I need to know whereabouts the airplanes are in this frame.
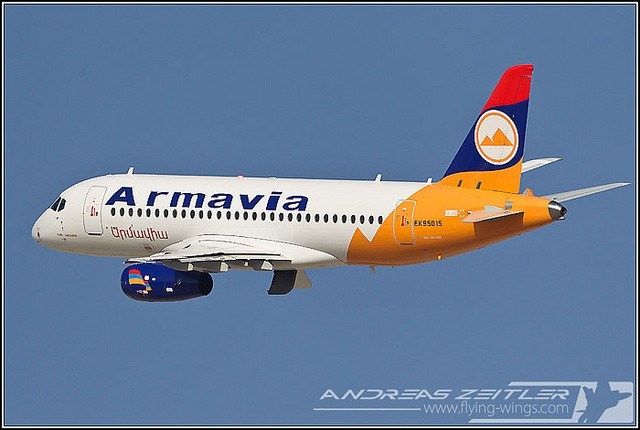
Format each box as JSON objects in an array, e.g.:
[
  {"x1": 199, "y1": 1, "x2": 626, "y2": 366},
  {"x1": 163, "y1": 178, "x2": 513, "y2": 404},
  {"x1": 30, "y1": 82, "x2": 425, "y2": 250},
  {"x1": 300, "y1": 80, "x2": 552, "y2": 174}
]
[{"x1": 31, "y1": 63, "x2": 631, "y2": 302}]
